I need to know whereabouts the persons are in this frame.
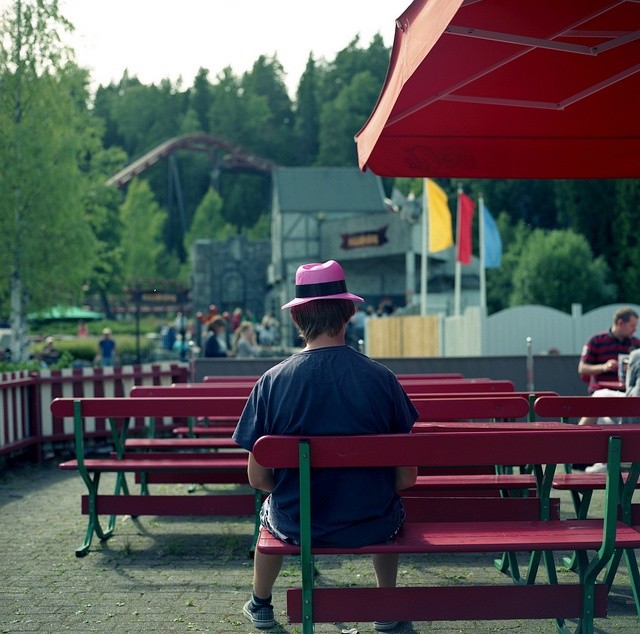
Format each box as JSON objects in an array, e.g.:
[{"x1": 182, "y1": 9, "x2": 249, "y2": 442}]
[
  {"x1": 579, "y1": 349, "x2": 640, "y2": 424},
  {"x1": 233, "y1": 322, "x2": 260, "y2": 358},
  {"x1": 352, "y1": 300, "x2": 406, "y2": 331},
  {"x1": 40, "y1": 336, "x2": 62, "y2": 364},
  {"x1": 579, "y1": 308, "x2": 640, "y2": 426},
  {"x1": 98, "y1": 328, "x2": 118, "y2": 366},
  {"x1": 232, "y1": 260, "x2": 421, "y2": 631},
  {"x1": 619, "y1": 349, "x2": 640, "y2": 425},
  {"x1": 206, "y1": 316, "x2": 235, "y2": 357}
]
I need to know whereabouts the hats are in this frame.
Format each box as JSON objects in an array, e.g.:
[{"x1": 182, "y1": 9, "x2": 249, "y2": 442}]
[{"x1": 281, "y1": 259, "x2": 365, "y2": 310}]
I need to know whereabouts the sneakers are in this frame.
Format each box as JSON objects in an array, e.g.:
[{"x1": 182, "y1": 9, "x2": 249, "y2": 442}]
[
  {"x1": 374, "y1": 620, "x2": 401, "y2": 630},
  {"x1": 242, "y1": 600, "x2": 276, "y2": 628},
  {"x1": 585, "y1": 463, "x2": 606, "y2": 473}
]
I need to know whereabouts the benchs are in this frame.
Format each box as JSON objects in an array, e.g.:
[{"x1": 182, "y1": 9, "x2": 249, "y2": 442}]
[
  {"x1": 203, "y1": 373, "x2": 465, "y2": 455},
  {"x1": 253, "y1": 427, "x2": 640, "y2": 633},
  {"x1": 168, "y1": 380, "x2": 519, "y2": 494},
  {"x1": 49, "y1": 395, "x2": 533, "y2": 586},
  {"x1": 129, "y1": 386, "x2": 560, "y2": 521},
  {"x1": 534, "y1": 395, "x2": 640, "y2": 573}
]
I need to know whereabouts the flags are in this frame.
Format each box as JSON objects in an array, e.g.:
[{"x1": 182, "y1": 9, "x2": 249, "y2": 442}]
[
  {"x1": 457, "y1": 194, "x2": 476, "y2": 265},
  {"x1": 483, "y1": 205, "x2": 503, "y2": 269},
  {"x1": 425, "y1": 177, "x2": 454, "y2": 254}
]
